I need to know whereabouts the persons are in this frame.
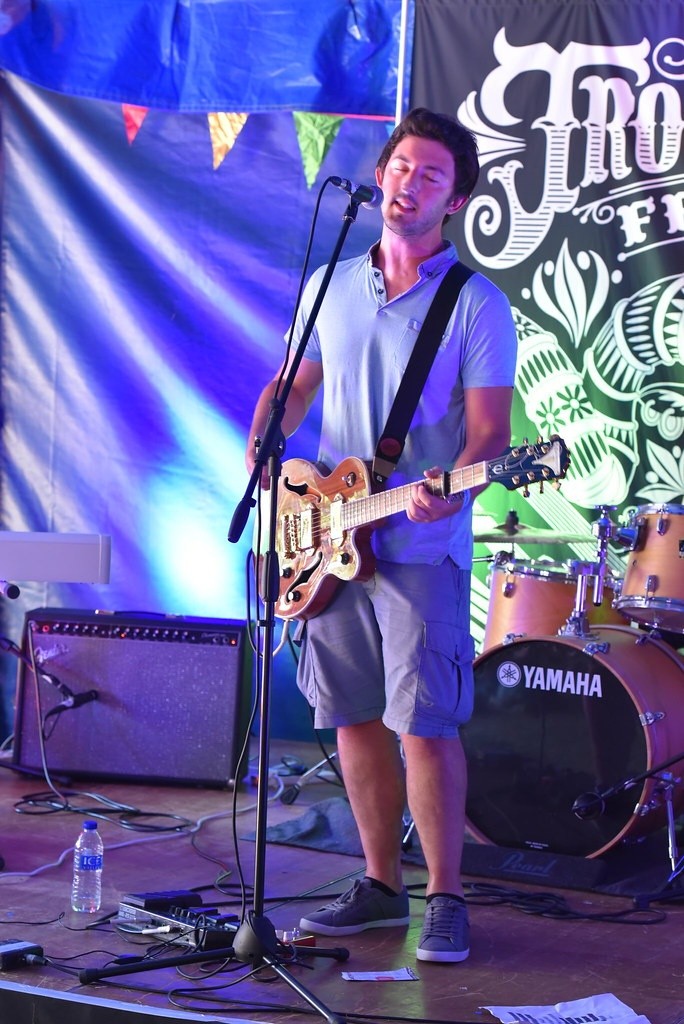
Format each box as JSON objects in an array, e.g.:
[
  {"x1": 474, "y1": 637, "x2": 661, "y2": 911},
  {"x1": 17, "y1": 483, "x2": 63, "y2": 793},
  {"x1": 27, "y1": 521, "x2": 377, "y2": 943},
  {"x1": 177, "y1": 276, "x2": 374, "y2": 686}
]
[{"x1": 247, "y1": 106, "x2": 518, "y2": 960}]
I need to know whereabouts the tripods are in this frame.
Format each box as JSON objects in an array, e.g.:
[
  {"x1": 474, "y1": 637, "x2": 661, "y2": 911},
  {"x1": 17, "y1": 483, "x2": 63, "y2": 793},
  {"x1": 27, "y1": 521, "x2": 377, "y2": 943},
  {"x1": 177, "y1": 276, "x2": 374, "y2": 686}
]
[{"x1": 77, "y1": 202, "x2": 350, "y2": 1024}]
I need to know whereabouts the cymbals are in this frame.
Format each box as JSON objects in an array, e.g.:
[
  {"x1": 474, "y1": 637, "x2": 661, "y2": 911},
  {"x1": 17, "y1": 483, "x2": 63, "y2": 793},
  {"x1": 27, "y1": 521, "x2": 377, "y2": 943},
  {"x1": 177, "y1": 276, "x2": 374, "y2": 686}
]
[{"x1": 473, "y1": 523, "x2": 599, "y2": 544}]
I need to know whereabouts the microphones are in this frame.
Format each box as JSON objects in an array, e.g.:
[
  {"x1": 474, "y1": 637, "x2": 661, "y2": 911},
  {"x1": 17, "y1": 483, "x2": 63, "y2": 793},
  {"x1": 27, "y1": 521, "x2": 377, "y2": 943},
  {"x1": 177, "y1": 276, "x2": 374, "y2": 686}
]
[
  {"x1": 331, "y1": 175, "x2": 384, "y2": 210},
  {"x1": 572, "y1": 792, "x2": 605, "y2": 820},
  {"x1": 0, "y1": 581, "x2": 21, "y2": 599},
  {"x1": 45, "y1": 690, "x2": 98, "y2": 719}
]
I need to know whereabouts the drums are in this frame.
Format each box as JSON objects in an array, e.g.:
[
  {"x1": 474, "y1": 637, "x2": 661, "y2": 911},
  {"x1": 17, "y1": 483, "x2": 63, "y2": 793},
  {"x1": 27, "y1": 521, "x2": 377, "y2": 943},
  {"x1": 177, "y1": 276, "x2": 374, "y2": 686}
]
[
  {"x1": 616, "y1": 502, "x2": 684, "y2": 634},
  {"x1": 480, "y1": 551, "x2": 631, "y2": 650},
  {"x1": 453, "y1": 624, "x2": 684, "y2": 860}
]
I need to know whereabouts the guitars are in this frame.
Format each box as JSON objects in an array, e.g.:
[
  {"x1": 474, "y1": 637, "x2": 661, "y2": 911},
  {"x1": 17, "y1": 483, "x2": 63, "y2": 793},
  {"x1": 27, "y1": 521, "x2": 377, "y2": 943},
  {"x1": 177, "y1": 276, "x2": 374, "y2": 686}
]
[{"x1": 249, "y1": 434, "x2": 569, "y2": 622}]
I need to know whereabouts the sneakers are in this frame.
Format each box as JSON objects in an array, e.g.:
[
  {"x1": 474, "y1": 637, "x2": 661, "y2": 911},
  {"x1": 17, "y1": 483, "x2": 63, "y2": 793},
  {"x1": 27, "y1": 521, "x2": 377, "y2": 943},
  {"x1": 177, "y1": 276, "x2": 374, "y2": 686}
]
[
  {"x1": 298, "y1": 877, "x2": 409, "y2": 936},
  {"x1": 416, "y1": 896, "x2": 471, "y2": 961}
]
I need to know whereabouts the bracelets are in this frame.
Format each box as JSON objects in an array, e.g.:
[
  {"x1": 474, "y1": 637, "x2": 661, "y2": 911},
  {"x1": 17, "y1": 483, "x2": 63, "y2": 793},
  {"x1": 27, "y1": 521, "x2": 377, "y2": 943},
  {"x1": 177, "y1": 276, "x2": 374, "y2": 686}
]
[{"x1": 462, "y1": 490, "x2": 471, "y2": 508}]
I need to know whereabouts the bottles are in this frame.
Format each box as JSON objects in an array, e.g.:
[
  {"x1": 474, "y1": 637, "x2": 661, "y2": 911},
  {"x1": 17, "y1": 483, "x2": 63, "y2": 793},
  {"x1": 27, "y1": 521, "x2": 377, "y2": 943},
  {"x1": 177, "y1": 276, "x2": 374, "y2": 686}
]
[{"x1": 71, "y1": 820, "x2": 102, "y2": 913}]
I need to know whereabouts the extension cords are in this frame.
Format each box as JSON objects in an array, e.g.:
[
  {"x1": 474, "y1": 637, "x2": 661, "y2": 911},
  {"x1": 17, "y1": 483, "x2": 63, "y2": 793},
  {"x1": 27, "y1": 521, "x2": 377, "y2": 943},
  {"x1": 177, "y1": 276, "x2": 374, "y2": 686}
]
[{"x1": 269, "y1": 769, "x2": 338, "y2": 786}]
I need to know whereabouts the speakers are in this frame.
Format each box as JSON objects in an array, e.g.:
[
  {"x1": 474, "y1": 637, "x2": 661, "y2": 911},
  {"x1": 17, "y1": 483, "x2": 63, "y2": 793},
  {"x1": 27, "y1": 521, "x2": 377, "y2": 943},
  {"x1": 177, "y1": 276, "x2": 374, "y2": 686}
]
[{"x1": 11, "y1": 606, "x2": 255, "y2": 787}]
